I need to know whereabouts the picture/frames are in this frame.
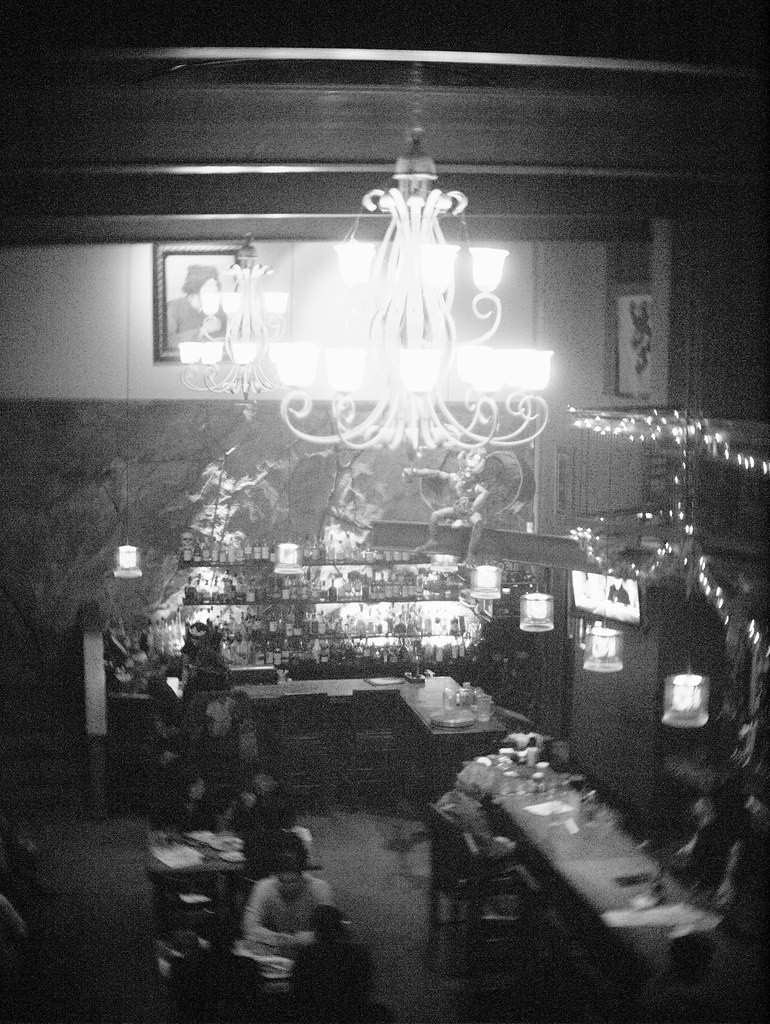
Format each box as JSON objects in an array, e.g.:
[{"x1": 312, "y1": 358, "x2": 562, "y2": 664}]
[
  {"x1": 603, "y1": 280, "x2": 655, "y2": 400},
  {"x1": 153, "y1": 239, "x2": 257, "y2": 363}
]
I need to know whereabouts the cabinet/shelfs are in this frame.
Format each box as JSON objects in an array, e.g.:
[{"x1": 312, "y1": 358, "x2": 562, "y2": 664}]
[{"x1": 170, "y1": 557, "x2": 490, "y2": 681}]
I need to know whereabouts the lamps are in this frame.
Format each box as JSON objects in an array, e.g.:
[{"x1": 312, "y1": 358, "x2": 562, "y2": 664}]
[{"x1": 111, "y1": 68, "x2": 708, "y2": 725}]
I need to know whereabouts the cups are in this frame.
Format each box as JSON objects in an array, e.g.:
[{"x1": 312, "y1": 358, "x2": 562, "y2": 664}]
[{"x1": 443, "y1": 682, "x2": 492, "y2": 722}]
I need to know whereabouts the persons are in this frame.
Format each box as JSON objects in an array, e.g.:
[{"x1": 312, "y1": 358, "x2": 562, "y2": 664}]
[
  {"x1": 207, "y1": 686, "x2": 239, "y2": 737},
  {"x1": 441, "y1": 792, "x2": 539, "y2": 916},
  {"x1": 241, "y1": 832, "x2": 322, "y2": 950},
  {"x1": 608, "y1": 578, "x2": 630, "y2": 604},
  {"x1": 239, "y1": 787, "x2": 321, "y2": 877},
  {"x1": 291, "y1": 904, "x2": 382, "y2": 1022},
  {"x1": 671, "y1": 795, "x2": 742, "y2": 911}
]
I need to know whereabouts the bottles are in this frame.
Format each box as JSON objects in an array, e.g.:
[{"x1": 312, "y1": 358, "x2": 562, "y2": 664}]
[{"x1": 147, "y1": 521, "x2": 481, "y2": 667}]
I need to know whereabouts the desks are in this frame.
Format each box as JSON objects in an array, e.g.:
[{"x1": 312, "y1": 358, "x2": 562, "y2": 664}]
[
  {"x1": 468, "y1": 753, "x2": 720, "y2": 983},
  {"x1": 398, "y1": 675, "x2": 504, "y2": 789},
  {"x1": 146, "y1": 830, "x2": 323, "y2": 936}
]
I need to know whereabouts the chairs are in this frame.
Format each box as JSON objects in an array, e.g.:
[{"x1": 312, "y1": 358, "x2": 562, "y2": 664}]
[{"x1": 423, "y1": 805, "x2": 535, "y2": 951}]
